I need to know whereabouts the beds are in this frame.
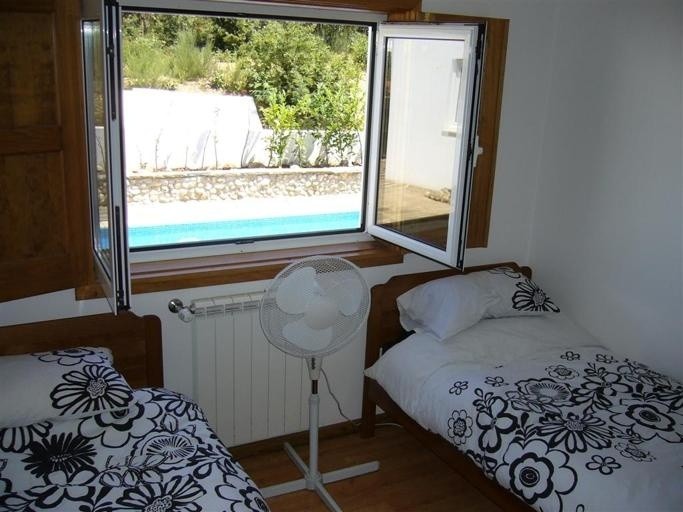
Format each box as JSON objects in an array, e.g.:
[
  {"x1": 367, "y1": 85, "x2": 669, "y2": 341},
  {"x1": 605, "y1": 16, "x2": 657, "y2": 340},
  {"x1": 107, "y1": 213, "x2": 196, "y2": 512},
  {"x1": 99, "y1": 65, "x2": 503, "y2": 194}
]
[
  {"x1": 0, "y1": 311, "x2": 271, "y2": 511},
  {"x1": 362, "y1": 262, "x2": 683, "y2": 512}
]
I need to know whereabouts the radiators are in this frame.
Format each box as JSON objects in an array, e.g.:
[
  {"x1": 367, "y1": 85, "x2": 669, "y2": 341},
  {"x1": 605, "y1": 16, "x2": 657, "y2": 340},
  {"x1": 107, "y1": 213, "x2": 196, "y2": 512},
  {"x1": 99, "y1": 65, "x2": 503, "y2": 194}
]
[{"x1": 168, "y1": 291, "x2": 367, "y2": 449}]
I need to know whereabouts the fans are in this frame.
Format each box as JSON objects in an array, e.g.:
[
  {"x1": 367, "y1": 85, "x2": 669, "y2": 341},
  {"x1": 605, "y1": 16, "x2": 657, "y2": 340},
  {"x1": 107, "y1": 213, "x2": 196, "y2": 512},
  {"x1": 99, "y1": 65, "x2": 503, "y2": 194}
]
[{"x1": 258, "y1": 255, "x2": 380, "y2": 512}]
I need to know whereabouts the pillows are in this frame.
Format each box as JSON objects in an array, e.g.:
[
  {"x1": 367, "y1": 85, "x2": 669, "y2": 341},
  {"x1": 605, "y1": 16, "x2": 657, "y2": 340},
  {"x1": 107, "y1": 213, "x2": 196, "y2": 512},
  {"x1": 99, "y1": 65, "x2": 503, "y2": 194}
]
[
  {"x1": 0, "y1": 346, "x2": 138, "y2": 431},
  {"x1": 395, "y1": 266, "x2": 561, "y2": 342}
]
